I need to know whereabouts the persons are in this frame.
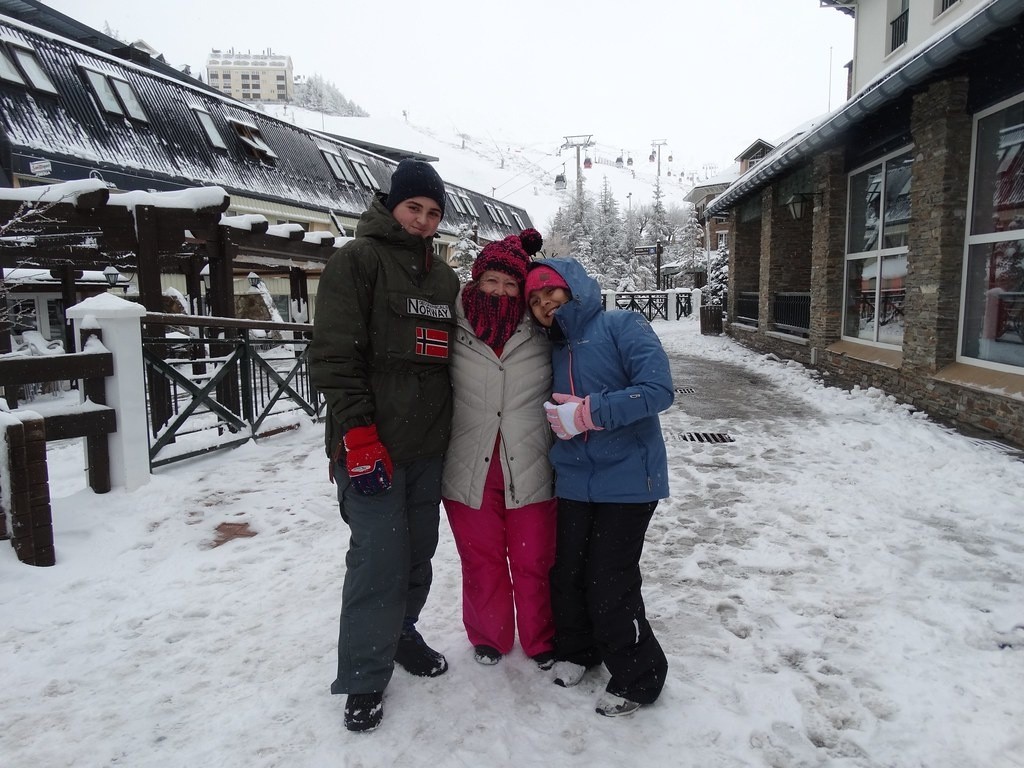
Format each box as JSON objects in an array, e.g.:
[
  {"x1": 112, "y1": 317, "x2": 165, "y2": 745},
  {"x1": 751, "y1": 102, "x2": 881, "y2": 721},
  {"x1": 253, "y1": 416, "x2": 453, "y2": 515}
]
[
  {"x1": 306, "y1": 160, "x2": 462, "y2": 734},
  {"x1": 520, "y1": 256, "x2": 677, "y2": 718},
  {"x1": 441, "y1": 226, "x2": 567, "y2": 670}
]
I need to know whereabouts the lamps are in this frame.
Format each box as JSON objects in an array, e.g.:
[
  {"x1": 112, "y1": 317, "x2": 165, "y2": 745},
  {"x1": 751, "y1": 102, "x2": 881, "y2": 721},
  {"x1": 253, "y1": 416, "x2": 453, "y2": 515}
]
[
  {"x1": 248, "y1": 272, "x2": 261, "y2": 289},
  {"x1": 102, "y1": 265, "x2": 120, "y2": 289},
  {"x1": 785, "y1": 191, "x2": 824, "y2": 221},
  {"x1": 200, "y1": 264, "x2": 211, "y2": 290}
]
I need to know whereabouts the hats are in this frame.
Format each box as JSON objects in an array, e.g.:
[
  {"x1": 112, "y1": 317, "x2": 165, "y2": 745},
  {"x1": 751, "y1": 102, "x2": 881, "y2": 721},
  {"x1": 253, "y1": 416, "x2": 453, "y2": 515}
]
[
  {"x1": 524, "y1": 266, "x2": 569, "y2": 304},
  {"x1": 386, "y1": 158, "x2": 445, "y2": 222},
  {"x1": 472, "y1": 228, "x2": 543, "y2": 281}
]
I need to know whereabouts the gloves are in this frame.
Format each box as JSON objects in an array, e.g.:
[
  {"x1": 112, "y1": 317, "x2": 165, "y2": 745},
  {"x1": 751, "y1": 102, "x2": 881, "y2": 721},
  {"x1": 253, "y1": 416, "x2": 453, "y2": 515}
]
[
  {"x1": 342, "y1": 424, "x2": 393, "y2": 496},
  {"x1": 543, "y1": 392, "x2": 604, "y2": 440}
]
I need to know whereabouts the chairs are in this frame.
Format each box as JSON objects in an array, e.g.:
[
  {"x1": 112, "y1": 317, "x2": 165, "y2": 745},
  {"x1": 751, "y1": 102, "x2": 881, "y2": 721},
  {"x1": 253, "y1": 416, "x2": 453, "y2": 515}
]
[{"x1": 10, "y1": 330, "x2": 65, "y2": 404}]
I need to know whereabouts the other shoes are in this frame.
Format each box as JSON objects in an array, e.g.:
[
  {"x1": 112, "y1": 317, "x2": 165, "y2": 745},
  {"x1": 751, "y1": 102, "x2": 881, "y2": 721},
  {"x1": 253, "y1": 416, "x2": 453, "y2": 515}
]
[
  {"x1": 552, "y1": 659, "x2": 588, "y2": 689},
  {"x1": 392, "y1": 627, "x2": 448, "y2": 680},
  {"x1": 473, "y1": 647, "x2": 501, "y2": 665},
  {"x1": 343, "y1": 687, "x2": 385, "y2": 733},
  {"x1": 594, "y1": 689, "x2": 643, "y2": 718},
  {"x1": 534, "y1": 653, "x2": 555, "y2": 671}
]
